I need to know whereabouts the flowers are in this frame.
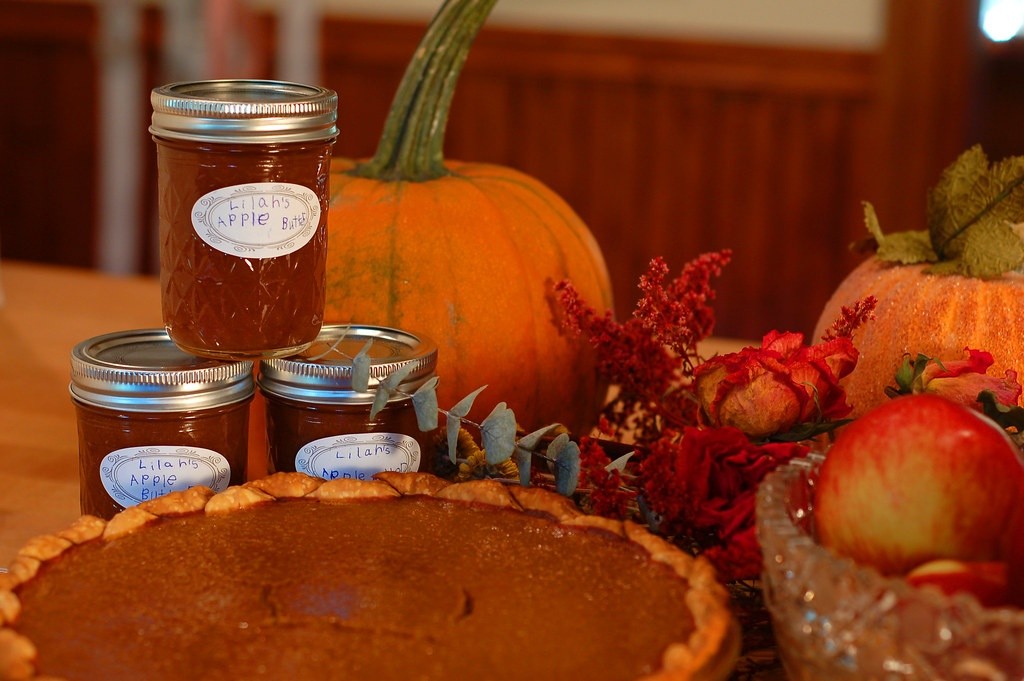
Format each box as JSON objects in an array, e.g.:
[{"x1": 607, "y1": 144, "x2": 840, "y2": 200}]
[{"x1": 308, "y1": 247, "x2": 1024, "y2": 619}]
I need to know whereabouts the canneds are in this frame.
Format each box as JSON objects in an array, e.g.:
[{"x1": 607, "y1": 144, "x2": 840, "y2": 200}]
[
  {"x1": 67, "y1": 326, "x2": 256, "y2": 522},
  {"x1": 147, "y1": 77, "x2": 342, "y2": 360},
  {"x1": 256, "y1": 322, "x2": 440, "y2": 480}
]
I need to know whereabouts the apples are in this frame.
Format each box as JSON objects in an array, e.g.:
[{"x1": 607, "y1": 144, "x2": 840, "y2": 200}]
[{"x1": 812, "y1": 390, "x2": 1024, "y2": 681}]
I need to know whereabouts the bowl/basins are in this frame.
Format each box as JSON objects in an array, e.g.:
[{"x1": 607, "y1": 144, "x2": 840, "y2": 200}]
[{"x1": 750, "y1": 443, "x2": 1022, "y2": 680}]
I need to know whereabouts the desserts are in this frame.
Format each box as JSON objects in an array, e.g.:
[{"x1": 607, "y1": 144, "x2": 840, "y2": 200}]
[{"x1": 0, "y1": 466, "x2": 740, "y2": 681}]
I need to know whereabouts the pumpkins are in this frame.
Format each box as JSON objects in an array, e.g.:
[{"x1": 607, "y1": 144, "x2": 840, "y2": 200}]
[
  {"x1": 246, "y1": 0, "x2": 616, "y2": 484},
  {"x1": 805, "y1": 250, "x2": 1024, "y2": 417}
]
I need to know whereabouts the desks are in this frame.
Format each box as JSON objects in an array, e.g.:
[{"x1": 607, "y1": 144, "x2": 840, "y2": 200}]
[{"x1": 0, "y1": 260, "x2": 774, "y2": 576}]
